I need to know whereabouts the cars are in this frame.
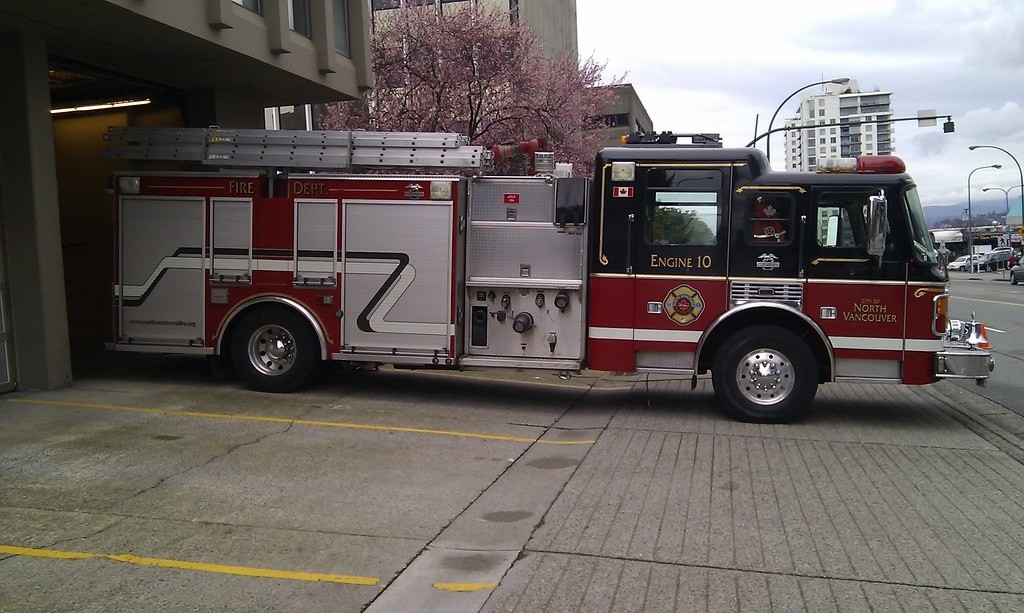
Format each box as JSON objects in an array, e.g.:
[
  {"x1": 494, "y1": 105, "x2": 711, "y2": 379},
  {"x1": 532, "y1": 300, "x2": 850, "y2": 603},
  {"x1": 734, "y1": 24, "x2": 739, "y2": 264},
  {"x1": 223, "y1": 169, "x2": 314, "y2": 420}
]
[
  {"x1": 1010, "y1": 256, "x2": 1024, "y2": 285},
  {"x1": 973, "y1": 254, "x2": 1007, "y2": 271},
  {"x1": 947, "y1": 255, "x2": 982, "y2": 272},
  {"x1": 985, "y1": 247, "x2": 1014, "y2": 254}
]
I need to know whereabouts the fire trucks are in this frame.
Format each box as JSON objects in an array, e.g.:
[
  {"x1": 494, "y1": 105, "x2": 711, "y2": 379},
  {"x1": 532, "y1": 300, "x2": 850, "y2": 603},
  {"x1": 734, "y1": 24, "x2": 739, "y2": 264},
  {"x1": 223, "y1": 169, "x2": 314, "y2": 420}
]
[{"x1": 104, "y1": 124, "x2": 994, "y2": 426}]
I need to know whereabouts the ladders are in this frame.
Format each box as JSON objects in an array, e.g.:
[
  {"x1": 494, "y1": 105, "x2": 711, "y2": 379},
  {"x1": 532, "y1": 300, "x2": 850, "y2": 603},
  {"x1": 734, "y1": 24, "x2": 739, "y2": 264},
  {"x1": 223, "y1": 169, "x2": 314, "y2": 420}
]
[{"x1": 101, "y1": 123, "x2": 497, "y2": 173}]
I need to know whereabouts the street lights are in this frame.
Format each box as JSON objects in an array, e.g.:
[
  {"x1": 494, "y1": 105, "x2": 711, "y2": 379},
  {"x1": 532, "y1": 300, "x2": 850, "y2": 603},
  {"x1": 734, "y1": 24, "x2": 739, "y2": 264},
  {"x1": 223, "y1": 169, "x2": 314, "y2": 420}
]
[
  {"x1": 983, "y1": 185, "x2": 1024, "y2": 257},
  {"x1": 766, "y1": 77, "x2": 851, "y2": 162},
  {"x1": 968, "y1": 165, "x2": 1002, "y2": 272},
  {"x1": 969, "y1": 146, "x2": 1024, "y2": 226}
]
